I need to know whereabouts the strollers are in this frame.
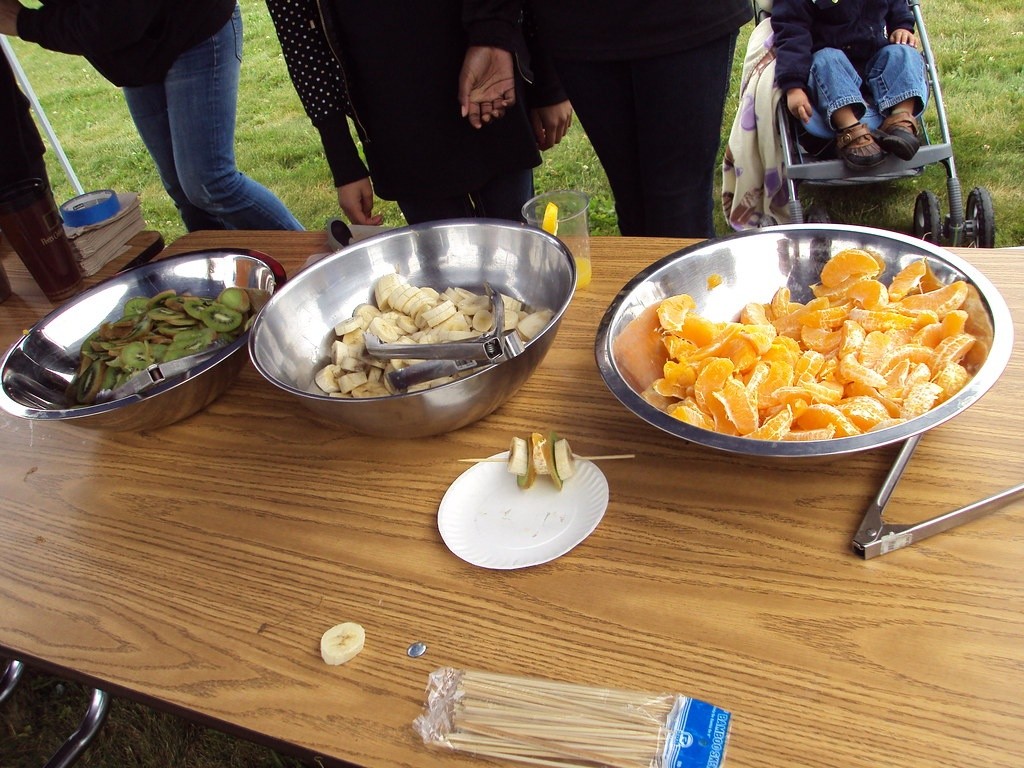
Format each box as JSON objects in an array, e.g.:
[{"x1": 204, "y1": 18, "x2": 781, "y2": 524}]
[{"x1": 756, "y1": 0, "x2": 999, "y2": 250}]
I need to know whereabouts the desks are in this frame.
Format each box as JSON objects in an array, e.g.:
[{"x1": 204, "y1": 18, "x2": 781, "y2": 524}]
[
  {"x1": 0, "y1": 229, "x2": 167, "y2": 366},
  {"x1": 0, "y1": 229, "x2": 1024, "y2": 766}
]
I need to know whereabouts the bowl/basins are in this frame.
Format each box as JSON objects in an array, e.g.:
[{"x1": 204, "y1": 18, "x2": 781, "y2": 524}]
[
  {"x1": 247, "y1": 216, "x2": 578, "y2": 441},
  {"x1": 0, "y1": 246, "x2": 288, "y2": 434},
  {"x1": 594, "y1": 222, "x2": 1016, "y2": 461}
]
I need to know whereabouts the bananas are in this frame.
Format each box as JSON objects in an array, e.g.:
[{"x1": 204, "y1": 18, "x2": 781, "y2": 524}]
[
  {"x1": 319, "y1": 621, "x2": 365, "y2": 665},
  {"x1": 315, "y1": 273, "x2": 553, "y2": 397},
  {"x1": 506, "y1": 437, "x2": 527, "y2": 476},
  {"x1": 554, "y1": 439, "x2": 576, "y2": 480}
]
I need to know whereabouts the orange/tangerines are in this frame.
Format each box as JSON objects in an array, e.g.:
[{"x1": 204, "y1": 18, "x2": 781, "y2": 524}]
[
  {"x1": 652, "y1": 248, "x2": 978, "y2": 442},
  {"x1": 531, "y1": 432, "x2": 549, "y2": 474}
]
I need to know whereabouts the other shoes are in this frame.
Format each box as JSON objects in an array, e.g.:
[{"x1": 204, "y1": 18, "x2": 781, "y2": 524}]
[
  {"x1": 837, "y1": 122, "x2": 887, "y2": 171},
  {"x1": 869, "y1": 111, "x2": 921, "y2": 161}
]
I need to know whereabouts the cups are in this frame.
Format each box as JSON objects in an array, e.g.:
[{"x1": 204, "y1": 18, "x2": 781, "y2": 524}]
[
  {"x1": 520, "y1": 190, "x2": 593, "y2": 291},
  {"x1": 0, "y1": 177, "x2": 84, "y2": 303}
]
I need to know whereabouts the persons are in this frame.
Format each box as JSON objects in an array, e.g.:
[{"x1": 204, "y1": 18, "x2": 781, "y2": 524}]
[
  {"x1": 0, "y1": 45, "x2": 49, "y2": 187},
  {"x1": 0, "y1": 0, "x2": 305, "y2": 233},
  {"x1": 458, "y1": 0, "x2": 755, "y2": 239},
  {"x1": 770, "y1": 0, "x2": 928, "y2": 173},
  {"x1": 265, "y1": 0, "x2": 573, "y2": 226}
]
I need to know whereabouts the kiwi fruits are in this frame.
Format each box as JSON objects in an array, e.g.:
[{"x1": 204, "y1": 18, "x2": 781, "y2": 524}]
[{"x1": 77, "y1": 287, "x2": 252, "y2": 409}]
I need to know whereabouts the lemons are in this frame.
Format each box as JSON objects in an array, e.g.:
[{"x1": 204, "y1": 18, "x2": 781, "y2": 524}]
[{"x1": 542, "y1": 202, "x2": 558, "y2": 236}]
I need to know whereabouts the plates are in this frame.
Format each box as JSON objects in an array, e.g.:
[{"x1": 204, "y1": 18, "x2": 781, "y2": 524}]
[{"x1": 437, "y1": 451, "x2": 610, "y2": 570}]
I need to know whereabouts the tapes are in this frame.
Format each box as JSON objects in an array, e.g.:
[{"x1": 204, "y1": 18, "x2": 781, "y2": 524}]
[{"x1": 59, "y1": 189, "x2": 121, "y2": 228}]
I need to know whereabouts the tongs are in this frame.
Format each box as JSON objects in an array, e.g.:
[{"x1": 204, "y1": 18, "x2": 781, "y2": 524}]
[
  {"x1": 108, "y1": 345, "x2": 227, "y2": 399},
  {"x1": 364, "y1": 277, "x2": 528, "y2": 393},
  {"x1": 849, "y1": 433, "x2": 1024, "y2": 561}
]
